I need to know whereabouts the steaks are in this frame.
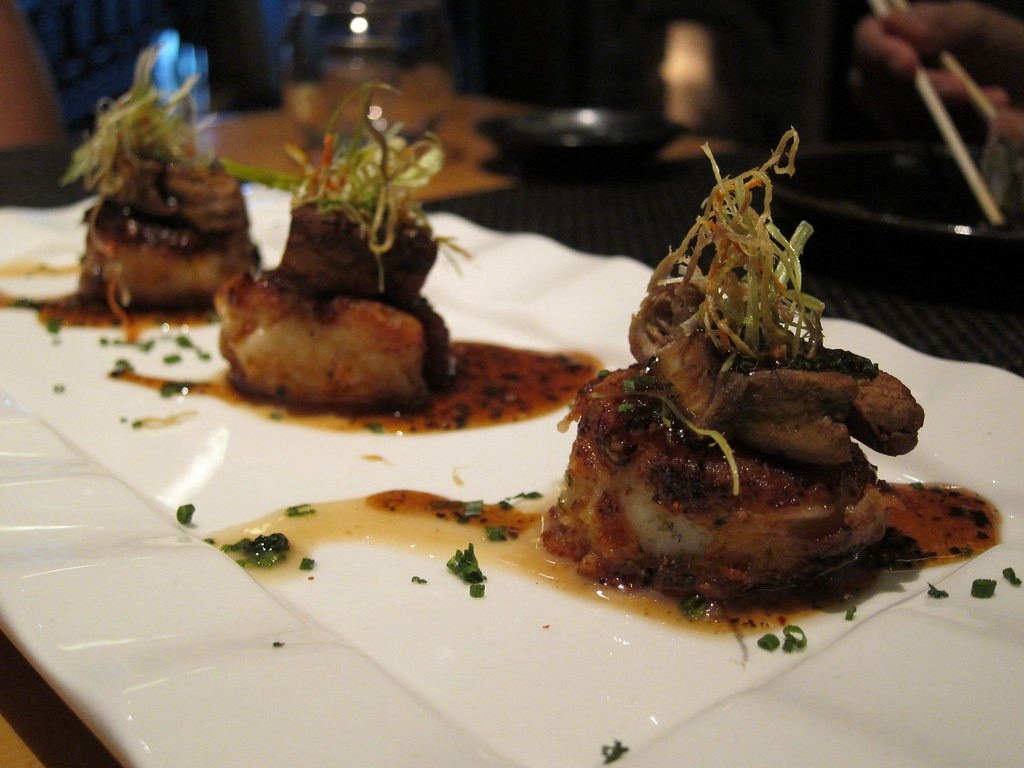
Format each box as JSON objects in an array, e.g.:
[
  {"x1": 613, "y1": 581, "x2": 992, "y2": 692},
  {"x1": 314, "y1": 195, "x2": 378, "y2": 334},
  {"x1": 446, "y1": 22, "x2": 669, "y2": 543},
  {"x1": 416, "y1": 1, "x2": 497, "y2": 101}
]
[
  {"x1": 540, "y1": 272, "x2": 926, "y2": 617},
  {"x1": 78, "y1": 164, "x2": 453, "y2": 412}
]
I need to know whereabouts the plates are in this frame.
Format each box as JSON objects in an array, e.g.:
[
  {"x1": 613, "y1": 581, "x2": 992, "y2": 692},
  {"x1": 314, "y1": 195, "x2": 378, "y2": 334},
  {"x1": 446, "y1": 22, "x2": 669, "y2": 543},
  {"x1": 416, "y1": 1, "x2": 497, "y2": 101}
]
[
  {"x1": 1, "y1": 183, "x2": 1023, "y2": 768},
  {"x1": 751, "y1": 141, "x2": 1024, "y2": 312},
  {"x1": 479, "y1": 107, "x2": 689, "y2": 177}
]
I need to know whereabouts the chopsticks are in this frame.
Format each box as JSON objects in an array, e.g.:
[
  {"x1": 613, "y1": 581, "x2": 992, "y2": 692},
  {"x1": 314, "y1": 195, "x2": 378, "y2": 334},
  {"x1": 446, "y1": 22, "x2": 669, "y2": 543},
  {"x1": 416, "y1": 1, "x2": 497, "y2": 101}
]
[{"x1": 864, "y1": 1, "x2": 1006, "y2": 226}]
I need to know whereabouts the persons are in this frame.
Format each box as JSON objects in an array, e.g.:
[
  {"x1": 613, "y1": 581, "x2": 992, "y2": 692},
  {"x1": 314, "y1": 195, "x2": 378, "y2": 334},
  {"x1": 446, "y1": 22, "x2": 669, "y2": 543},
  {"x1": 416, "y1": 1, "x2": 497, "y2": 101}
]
[
  {"x1": 9, "y1": 1, "x2": 259, "y2": 145},
  {"x1": 844, "y1": 1, "x2": 1024, "y2": 223},
  {"x1": 258, "y1": 1, "x2": 442, "y2": 107}
]
